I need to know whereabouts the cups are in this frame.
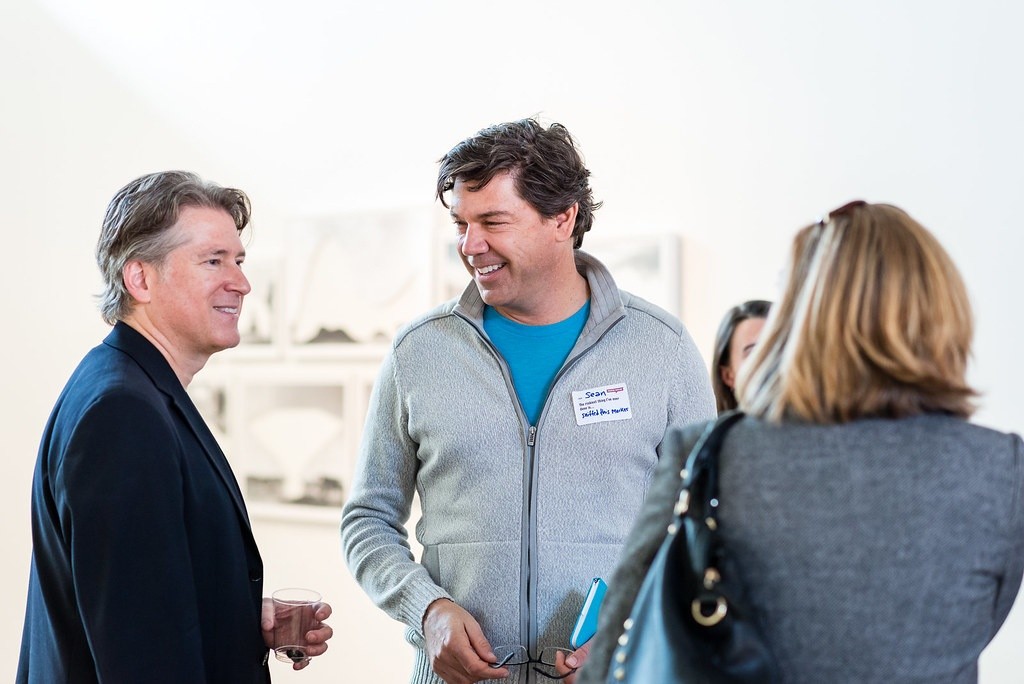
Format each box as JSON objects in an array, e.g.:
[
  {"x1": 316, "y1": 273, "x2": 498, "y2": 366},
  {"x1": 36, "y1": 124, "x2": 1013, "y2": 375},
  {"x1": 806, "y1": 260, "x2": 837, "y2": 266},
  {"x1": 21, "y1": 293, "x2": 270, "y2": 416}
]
[{"x1": 272, "y1": 588, "x2": 321, "y2": 662}]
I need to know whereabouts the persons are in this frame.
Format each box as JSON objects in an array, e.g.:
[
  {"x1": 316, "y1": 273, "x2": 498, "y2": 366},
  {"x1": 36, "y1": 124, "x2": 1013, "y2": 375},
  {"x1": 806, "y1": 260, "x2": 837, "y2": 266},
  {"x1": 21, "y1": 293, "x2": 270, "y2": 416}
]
[
  {"x1": 16, "y1": 171, "x2": 332, "y2": 684},
  {"x1": 340, "y1": 120, "x2": 1024, "y2": 682}
]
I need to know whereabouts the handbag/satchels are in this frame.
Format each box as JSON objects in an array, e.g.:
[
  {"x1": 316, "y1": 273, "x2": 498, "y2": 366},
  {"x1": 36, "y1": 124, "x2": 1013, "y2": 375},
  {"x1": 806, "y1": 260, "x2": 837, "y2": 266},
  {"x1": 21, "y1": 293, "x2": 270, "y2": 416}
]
[{"x1": 604, "y1": 412, "x2": 786, "y2": 684}]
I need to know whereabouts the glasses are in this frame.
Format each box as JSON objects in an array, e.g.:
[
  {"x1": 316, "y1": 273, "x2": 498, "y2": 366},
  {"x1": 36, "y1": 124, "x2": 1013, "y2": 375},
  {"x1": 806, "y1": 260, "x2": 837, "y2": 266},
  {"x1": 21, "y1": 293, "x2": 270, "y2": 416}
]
[
  {"x1": 819, "y1": 200, "x2": 868, "y2": 226},
  {"x1": 489, "y1": 645, "x2": 579, "y2": 679}
]
